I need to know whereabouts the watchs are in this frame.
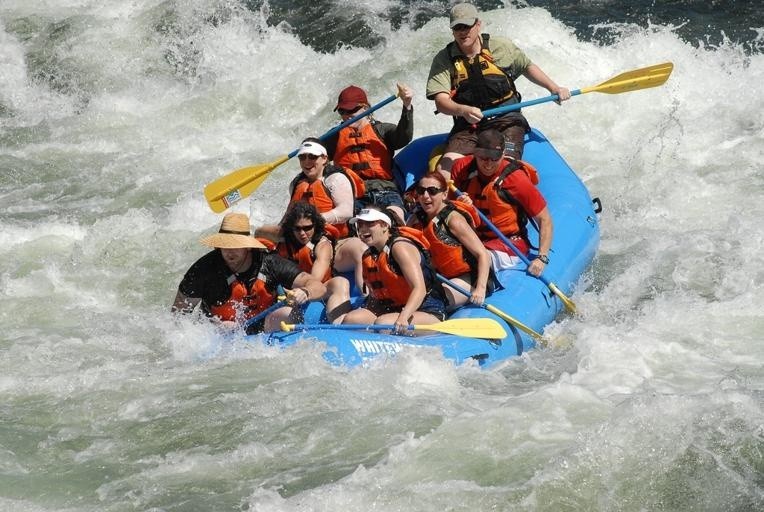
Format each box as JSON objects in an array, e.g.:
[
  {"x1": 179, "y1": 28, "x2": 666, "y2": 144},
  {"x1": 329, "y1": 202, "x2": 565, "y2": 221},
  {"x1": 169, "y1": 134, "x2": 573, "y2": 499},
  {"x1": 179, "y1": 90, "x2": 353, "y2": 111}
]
[{"x1": 536, "y1": 255, "x2": 549, "y2": 265}]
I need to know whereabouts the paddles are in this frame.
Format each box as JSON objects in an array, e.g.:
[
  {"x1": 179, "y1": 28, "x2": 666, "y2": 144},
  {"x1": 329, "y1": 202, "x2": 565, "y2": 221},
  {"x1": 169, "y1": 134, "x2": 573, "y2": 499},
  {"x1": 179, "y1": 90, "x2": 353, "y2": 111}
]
[
  {"x1": 447, "y1": 180, "x2": 577, "y2": 314},
  {"x1": 279, "y1": 318, "x2": 507, "y2": 339},
  {"x1": 203, "y1": 82, "x2": 403, "y2": 212},
  {"x1": 480, "y1": 62, "x2": 673, "y2": 118},
  {"x1": 421, "y1": 258, "x2": 551, "y2": 347}
]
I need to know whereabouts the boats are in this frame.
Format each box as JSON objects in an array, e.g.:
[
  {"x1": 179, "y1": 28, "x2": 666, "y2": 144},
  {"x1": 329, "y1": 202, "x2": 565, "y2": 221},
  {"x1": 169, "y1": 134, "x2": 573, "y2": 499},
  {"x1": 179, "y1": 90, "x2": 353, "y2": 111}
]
[{"x1": 196, "y1": 122, "x2": 604, "y2": 380}]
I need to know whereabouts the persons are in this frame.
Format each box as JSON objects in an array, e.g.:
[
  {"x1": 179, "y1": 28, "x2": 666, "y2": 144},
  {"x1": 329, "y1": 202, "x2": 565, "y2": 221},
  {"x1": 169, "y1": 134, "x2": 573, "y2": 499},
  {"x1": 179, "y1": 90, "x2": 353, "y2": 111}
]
[
  {"x1": 447, "y1": 129, "x2": 553, "y2": 277},
  {"x1": 270, "y1": 200, "x2": 352, "y2": 327},
  {"x1": 171, "y1": 213, "x2": 328, "y2": 334},
  {"x1": 319, "y1": 84, "x2": 414, "y2": 228},
  {"x1": 343, "y1": 207, "x2": 447, "y2": 337},
  {"x1": 255, "y1": 138, "x2": 371, "y2": 296},
  {"x1": 403, "y1": 171, "x2": 492, "y2": 313},
  {"x1": 425, "y1": 3, "x2": 571, "y2": 163}
]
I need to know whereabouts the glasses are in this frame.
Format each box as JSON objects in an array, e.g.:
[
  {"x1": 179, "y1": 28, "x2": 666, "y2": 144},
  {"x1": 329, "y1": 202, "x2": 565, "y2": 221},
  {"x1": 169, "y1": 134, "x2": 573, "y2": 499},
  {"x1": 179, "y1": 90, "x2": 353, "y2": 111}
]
[
  {"x1": 338, "y1": 106, "x2": 362, "y2": 115},
  {"x1": 291, "y1": 224, "x2": 314, "y2": 231},
  {"x1": 298, "y1": 154, "x2": 318, "y2": 160},
  {"x1": 416, "y1": 187, "x2": 441, "y2": 194}
]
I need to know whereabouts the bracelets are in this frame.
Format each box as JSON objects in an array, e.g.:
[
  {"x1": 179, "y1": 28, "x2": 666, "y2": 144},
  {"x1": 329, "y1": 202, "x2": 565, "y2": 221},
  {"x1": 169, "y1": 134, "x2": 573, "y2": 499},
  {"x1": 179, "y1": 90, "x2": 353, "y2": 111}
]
[{"x1": 301, "y1": 288, "x2": 309, "y2": 298}]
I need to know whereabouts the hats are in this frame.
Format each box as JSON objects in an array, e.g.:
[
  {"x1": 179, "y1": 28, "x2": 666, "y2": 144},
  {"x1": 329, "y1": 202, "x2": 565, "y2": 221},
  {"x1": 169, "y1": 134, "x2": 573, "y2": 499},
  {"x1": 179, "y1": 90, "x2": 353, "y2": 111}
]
[
  {"x1": 450, "y1": 3, "x2": 478, "y2": 28},
  {"x1": 349, "y1": 209, "x2": 392, "y2": 228},
  {"x1": 200, "y1": 213, "x2": 268, "y2": 249},
  {"x1": 333, "y1": 86, "x2": 367, "y2": 112},
  {"x1": 296, "y1": 142, "x2": 327, "y2": 157},
  {"x1": 474, "y1": 130, "x2": 504, "y2": 160}
]
